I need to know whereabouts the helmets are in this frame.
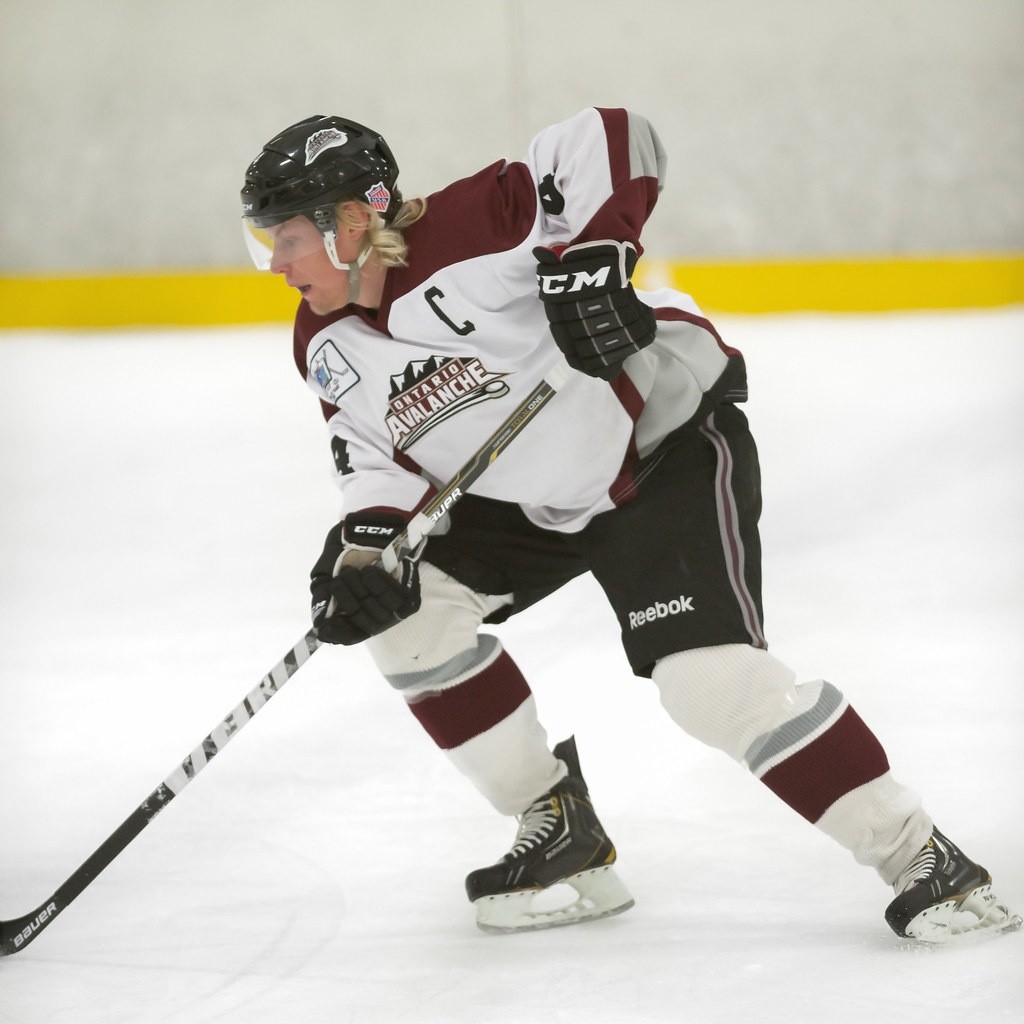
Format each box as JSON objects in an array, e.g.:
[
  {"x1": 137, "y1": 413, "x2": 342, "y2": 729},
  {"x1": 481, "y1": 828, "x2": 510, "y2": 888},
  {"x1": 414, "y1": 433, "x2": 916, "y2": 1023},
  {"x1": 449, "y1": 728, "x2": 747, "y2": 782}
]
[{"x1": 240, "y1": 112, "x2": 404, "y2": 269}]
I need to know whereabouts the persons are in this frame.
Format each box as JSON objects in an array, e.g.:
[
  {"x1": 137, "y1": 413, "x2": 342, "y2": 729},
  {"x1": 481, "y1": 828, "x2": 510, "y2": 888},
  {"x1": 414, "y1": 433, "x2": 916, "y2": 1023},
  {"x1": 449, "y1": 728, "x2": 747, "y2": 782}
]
[{"x1": 236, "y1": 108, "x2": 1023, "y2": 951}]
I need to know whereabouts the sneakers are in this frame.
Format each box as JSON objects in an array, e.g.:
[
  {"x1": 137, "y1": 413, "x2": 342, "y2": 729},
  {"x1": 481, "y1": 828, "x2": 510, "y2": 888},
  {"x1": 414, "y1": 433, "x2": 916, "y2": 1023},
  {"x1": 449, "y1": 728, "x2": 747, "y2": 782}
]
[
  {"x1": 885, "y1": 824, "x2": 1024, "y2": 947},
  {"x1": 460, "y1": 733, "x2": 633, "y2": 935}
]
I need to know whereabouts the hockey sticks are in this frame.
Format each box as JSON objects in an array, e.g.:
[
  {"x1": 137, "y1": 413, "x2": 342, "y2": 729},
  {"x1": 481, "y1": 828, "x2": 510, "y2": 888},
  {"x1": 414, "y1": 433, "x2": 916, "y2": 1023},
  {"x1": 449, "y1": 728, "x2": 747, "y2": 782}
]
[{"x1": 0, "y1": 354, "x2": 580, "y2": 958}]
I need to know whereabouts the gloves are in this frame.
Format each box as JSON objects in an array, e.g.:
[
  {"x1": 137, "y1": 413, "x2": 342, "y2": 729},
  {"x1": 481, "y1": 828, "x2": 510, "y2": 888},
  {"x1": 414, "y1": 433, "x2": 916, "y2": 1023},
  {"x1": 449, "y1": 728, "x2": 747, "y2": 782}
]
[
  {"x1": 531, "y1": 240, "x2": 656, "y2": 380},
  {"x1": 309, "y1": 511, "x2": 420, "y2": 646}
]
